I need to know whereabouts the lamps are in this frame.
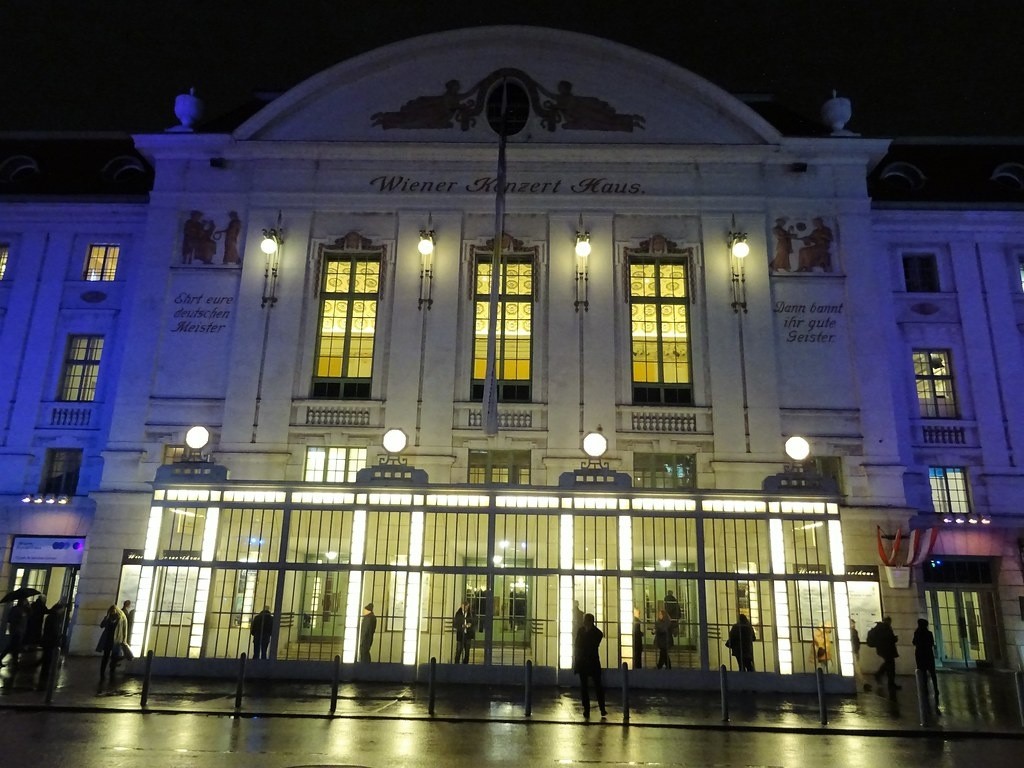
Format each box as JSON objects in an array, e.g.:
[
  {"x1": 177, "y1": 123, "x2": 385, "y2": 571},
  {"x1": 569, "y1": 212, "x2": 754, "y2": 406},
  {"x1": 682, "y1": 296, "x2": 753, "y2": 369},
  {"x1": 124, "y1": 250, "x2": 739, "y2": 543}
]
[
  {"x1": 784, "y1": 436, "x2": 820, "y2": 472},
  {"x1": 181, "y1": 425, "x2": 210, "y2": 462},
  {"x1": 21, "y1": 493, "x2": 69, "y2": 505},
  {"x1": 582, "y1": 432, "x2": 610, "y2": 470},
  {"x1": 418, "y1": 212, "x2": 436, "y2": 311},
  {"x1": 379, "y1": 428, "x2": 408, "y2": 465},
  {"x1": 574, "y1": 213, "x2": 592, "y2": 313},
  {"x1": 261, "y1": 209, "x2": 284, "y2": 308},
  {"x1": 728, "y1": 212, "x2": 749, "y2": 313}
]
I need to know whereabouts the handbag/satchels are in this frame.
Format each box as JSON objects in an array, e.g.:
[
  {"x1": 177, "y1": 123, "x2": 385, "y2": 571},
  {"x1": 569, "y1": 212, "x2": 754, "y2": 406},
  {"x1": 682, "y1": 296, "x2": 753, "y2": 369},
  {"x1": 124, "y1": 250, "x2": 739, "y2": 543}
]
[{"x1": 122, "y1": 643, "x2": 134, "y2": 660}]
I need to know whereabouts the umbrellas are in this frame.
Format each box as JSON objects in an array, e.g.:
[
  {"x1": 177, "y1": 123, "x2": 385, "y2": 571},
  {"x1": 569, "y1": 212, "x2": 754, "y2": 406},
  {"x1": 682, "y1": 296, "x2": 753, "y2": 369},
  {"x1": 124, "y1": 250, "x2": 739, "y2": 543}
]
[{"x1": 0, "y1": 587, "x2": 42, "y2": 604}]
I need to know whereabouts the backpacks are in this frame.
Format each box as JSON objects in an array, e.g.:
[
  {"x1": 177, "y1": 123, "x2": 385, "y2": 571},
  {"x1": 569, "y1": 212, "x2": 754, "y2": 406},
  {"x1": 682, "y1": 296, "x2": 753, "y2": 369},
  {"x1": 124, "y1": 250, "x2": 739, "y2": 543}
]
[{"x1": 867, "y1": 622, "x2": 890, "y2": 647}]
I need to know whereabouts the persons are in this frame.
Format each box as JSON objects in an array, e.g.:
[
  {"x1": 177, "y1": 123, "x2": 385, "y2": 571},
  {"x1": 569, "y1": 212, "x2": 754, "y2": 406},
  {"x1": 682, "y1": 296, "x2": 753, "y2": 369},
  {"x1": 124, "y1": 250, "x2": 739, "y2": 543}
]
[
  {"x1": 850, "y1": 619, "x2": 873, "y2": 690},
  {"x1": 250, "y1": 605, "x2": 273, "y2": 660},
  {"x1": 0, "y1": 595, "x2": 72, "y2": 672},
  {"x1": 872, "y1": 617, "x2": 903, "y2": 691},
  {"x1": 807, "y1": 620, "x2": 836, "y2": 674},
  {"x1": 219, "y1": 210, "x2": 242, "y2": 265},
  {"x1": 117, "y1": 600, "x2": 134, "y2": 666},
  {"x1": 633, "y1": 607, "x2": 645, "y2": 671},
  {"x1": 794, "y1": 217, "x2": 835, "y2": 272},
  {"x1": 182, "y1": 208, "x2": 217, "y2": 265},
  {"x1": 370, "y1": 77, "x2": 480, "y2": 129},
  {"x1": 770, "y1": 217, "x2": 798, "y2": 272},
  {"x1": 725, "y1": 614, "x2": 758, "y2": 672},
  {"x1": 535, "y1": 79, "x2": 647, "y2": 134},
  {"x1": 573, "y1": 612, "x2": 609, "y2": 719},
  {"x1": 663, "y1": 589, "x2": 682, "y2": 638},
  {"x1": 452, "y1": 601, "x2": 477, "y2": 665},
  {"x1": 359, "y1": 602, "x2": 378, "y2": 663},
  {"x1": 574, "y1": 600, "x2": 584, "y2": 629},
  {"x1": 94, "y1": 605, "x2": 129, "y2": 695},
  {"x1": 911, "y1": 618, "x2": 940, "y2": 696},
  {"x1": 651, "y1": 610, "x2": 679, "y2": 671}
]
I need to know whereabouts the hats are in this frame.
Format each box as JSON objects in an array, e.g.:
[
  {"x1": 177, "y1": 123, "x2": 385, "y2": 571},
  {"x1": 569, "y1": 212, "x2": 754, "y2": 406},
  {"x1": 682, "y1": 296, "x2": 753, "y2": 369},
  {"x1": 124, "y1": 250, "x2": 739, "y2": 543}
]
[{"x1": 365, "y1": 603, "x2": 373, "y2": 611}]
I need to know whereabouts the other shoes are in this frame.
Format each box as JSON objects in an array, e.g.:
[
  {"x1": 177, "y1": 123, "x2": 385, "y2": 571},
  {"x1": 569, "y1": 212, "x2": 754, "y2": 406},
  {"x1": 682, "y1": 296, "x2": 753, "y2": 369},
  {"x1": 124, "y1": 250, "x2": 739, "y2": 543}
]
[
  {"x1": 601, "y1": 711, "x2": 607, "y2": 716},
  {"x1": 886, "y1": 682, "x2": 902, "y2": 690},
  {"x1": 874, "y1": 672, "x2": 881, "y2": 682},
  {"x1": 934, "y1": 687, "x2": 939, "y2": 695},
  {"x1": 584, "y1": 711, "x2": 590, "y2": 717}
]
